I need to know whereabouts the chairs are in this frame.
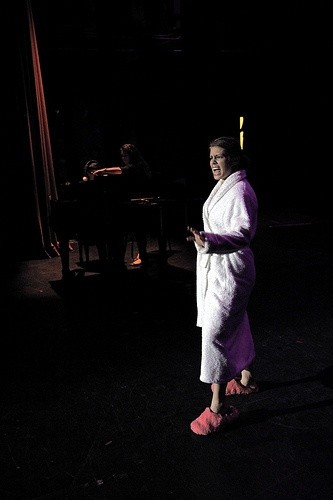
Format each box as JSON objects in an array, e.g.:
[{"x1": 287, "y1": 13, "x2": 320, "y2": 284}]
[{"x1": 74, "y1": 227, "x2": 110, "y2": 265}]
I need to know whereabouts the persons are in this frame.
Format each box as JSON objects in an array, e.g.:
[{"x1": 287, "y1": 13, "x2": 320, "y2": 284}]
[{"x1": 188, "y1": 137, "x2": 258, "y2": 435}]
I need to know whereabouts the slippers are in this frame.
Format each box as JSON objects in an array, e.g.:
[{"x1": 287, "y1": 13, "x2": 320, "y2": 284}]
[
  {"x1": 211, "y1": 374, "x2": 259, "y2": 396},
  {"x1": 191, "y1": 402, "x2": 239, "y2": 436}
]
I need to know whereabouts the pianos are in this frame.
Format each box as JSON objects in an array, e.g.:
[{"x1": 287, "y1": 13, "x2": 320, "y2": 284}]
[{"x1": 49, "y1": 173, "x2": 185, "y2": 278}]
